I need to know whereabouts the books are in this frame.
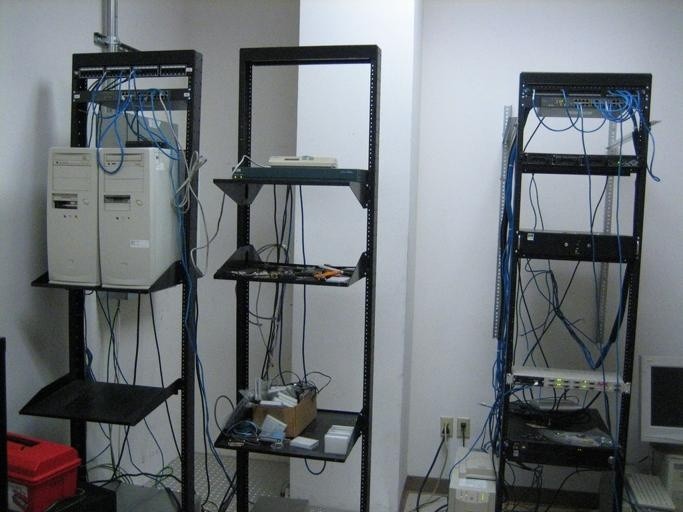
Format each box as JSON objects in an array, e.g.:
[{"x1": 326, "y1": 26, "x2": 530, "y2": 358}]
[
  {"x1": 323, "y1": 424, "x2": 353, "y2": 456},
  {"x1": 254, "y1": 376, "x2": 299, "y2": 409},
  {"x1": 257, "y1": 437, "x2": 284, "y2": 444},
  {"x1": 289, "y1": 445, "x2": 320, "y2": 451},
  {"x1": 259, "y1": 414, "x2": 287, "y2": 434},
  {"x1": 289, "y1": 435, "x2": 319, "y2": 447}
]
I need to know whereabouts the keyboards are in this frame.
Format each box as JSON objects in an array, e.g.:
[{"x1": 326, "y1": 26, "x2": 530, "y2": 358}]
[{"x1": 627, "y1": 471, "x2": 674, "y2": 510}]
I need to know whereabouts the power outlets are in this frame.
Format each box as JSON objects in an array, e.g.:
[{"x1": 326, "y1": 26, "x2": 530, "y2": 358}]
[
  {"x1": 439, "y1": 418, "x2": 454, "y2": 437},
  {"x1": 456, "y1": 417, "x2": 470, "y2": 439}
]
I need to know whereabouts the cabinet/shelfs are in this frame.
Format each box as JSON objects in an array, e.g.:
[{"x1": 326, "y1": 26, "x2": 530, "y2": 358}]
[
  {"x1": 19, "y1": 44, "x2": 201, "y2": 512},
  {"x1": 494, "y1": 69, "x2": 653, "y2": 512},
  {"x1": 214, "y1": 45, "x2": 377, "y2": 512}
]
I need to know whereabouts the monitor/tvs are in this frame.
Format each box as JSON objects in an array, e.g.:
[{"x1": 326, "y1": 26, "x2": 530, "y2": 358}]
[{"x1": 640, "y1": 354, "x2": 682, "y2": 447}]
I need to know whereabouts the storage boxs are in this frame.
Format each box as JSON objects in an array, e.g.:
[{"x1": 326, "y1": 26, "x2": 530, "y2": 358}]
[{"x1": 253, "y1": 389, "x2": 316, "y2": 438}]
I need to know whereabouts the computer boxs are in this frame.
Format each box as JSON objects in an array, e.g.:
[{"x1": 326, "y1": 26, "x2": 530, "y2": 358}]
[
  {"x1": 98, "y1": 147, "x2": 184, "y2": 290},
  {"x1": 446, "y1": 447, "x2": 495, "y2": 512},
  {"x1": 46, "y1": 146, "x2": 100, "y2": 291}
]
